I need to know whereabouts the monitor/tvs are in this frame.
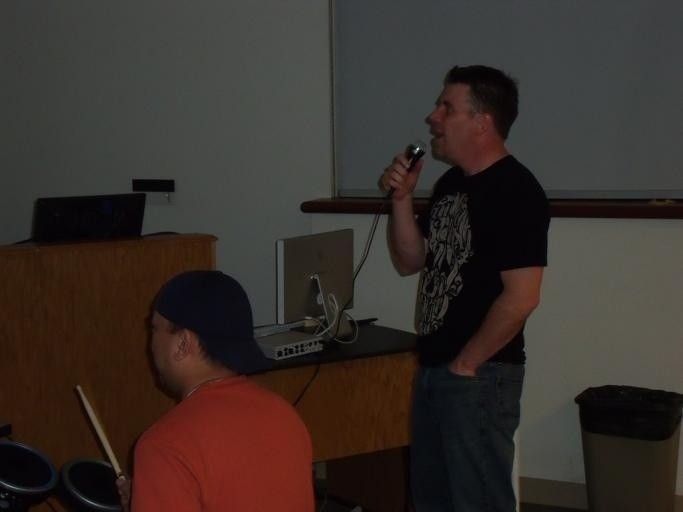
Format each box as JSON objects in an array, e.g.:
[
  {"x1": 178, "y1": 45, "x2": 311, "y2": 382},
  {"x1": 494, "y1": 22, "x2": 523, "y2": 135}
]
[
  {"x1": 34, "y1": 193, "x2": 146, "y2": 243},
  {"x1": 276, "y1": 229, "x2": 354, "y2": 342}
]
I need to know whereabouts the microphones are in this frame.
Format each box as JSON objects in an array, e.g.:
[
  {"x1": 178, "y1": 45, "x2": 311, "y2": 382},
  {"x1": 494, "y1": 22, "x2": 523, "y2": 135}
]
[{"x1": 386, "y1": 140, "x2": 427, "y2": 197}]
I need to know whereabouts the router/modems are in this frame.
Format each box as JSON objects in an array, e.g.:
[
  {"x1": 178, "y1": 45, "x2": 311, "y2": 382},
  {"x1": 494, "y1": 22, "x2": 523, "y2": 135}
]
[{"x1": 257, "y1": 331, "x2": 323, "y2": 360}]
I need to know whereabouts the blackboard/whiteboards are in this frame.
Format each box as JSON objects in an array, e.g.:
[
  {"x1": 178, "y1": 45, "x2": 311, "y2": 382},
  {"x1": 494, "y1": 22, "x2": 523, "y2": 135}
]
[{"x1": 329, "y1": 1, "x2": 680, "y2": 200}]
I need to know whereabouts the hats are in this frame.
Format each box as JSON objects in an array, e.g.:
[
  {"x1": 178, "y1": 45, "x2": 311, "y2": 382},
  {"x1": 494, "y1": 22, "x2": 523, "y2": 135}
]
[{"x1": 151, "y1": 270, "x2": 278, "y2": 374}]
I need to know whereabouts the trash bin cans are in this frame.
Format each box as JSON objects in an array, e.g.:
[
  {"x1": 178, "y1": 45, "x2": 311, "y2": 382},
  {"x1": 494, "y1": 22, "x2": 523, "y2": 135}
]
[{"x1": 574, "y1": 382, "x2": 683, "y2": 510}]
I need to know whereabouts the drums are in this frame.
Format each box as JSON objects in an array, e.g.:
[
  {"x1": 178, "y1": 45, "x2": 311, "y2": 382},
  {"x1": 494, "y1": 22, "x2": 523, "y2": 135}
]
[
  {"x1": 57, "y1": 459, "x2": 130, "y2": 511},
  {"x1": 0, "y1": 439, "x2": 57, "y2": 512}
]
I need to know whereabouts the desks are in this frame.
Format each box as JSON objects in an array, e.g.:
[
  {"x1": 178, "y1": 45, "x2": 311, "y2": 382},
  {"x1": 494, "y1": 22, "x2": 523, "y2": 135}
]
[{"x1": 244, "y1": 322, "x2": 423, "y2": 512}]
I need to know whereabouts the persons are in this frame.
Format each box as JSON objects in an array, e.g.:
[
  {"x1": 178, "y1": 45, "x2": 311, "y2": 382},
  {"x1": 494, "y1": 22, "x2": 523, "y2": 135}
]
[
  {"x1": 115, "y1": 269, "x2": 318, "y2": 512},
  {"x1": 378, "y1": 65, "x2": 551, "y2": 510}
]
